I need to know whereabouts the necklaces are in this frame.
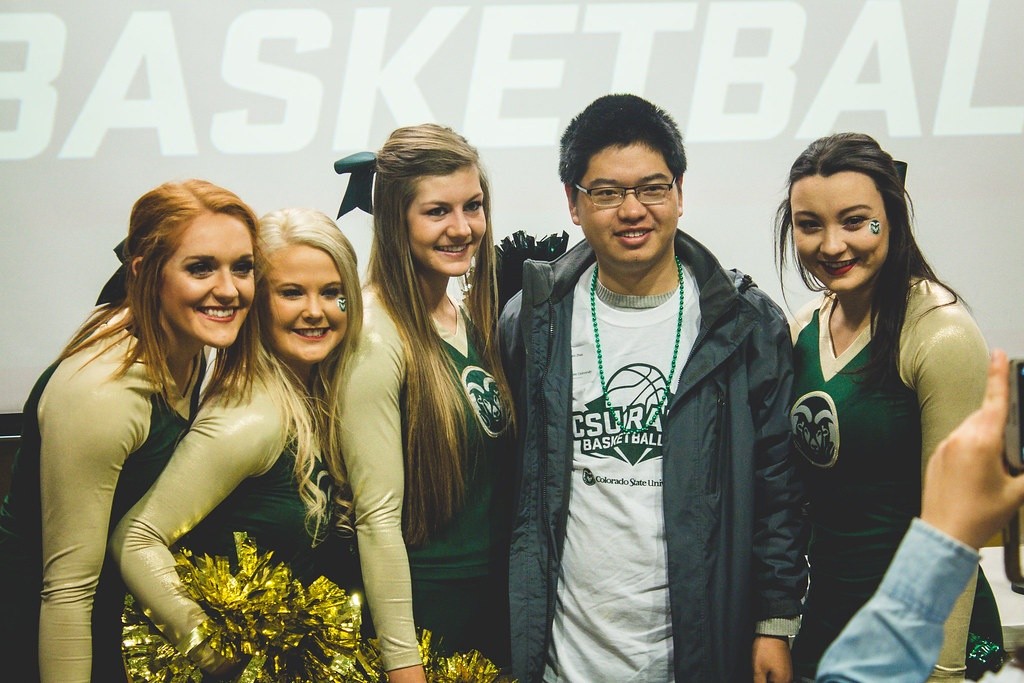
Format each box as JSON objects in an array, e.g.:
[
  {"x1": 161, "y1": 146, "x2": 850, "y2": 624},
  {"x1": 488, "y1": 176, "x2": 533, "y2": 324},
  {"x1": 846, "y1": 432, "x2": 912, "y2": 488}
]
[{"x1": 590, "y1": 256, "x2": 684, "y2": 433}]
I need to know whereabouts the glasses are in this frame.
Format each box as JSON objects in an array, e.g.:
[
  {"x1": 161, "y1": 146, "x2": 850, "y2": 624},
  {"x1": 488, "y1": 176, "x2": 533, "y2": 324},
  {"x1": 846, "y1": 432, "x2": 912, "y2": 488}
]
[{"x1": 575, "y1": 175, "x2": 676, "y2": 209}]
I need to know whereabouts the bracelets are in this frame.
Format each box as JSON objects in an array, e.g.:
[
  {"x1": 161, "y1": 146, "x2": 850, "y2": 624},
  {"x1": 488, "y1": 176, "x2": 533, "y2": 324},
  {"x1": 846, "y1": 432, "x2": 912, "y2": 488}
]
[{"x1": 934, "y1": 665, "x2": 967, "y2": 672}]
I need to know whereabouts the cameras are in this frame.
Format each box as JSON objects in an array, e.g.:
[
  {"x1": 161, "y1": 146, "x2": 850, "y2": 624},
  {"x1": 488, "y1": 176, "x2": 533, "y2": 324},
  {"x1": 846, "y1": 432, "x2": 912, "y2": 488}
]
[{"x1": 1003, "y1": 359, "x2": 1024, "y2": 471}]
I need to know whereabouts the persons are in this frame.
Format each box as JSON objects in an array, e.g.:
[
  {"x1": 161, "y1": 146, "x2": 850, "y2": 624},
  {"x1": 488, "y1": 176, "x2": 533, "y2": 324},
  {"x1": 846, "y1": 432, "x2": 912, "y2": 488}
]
[
  {"x1": 497, "y1": 93, "x2": 809, "y2": 683},
  {"x1": 773, "y1": 132, "x2": 1003, "y2": 683},
  {"x1": 110, "y1": 209, "x2": 376, "y2": 683},
  {"x1": 0, "y1": 179, "x2": 261, "y2": 683},
  {"x1": 815, "y1": 349, "x2": 1024, "y2": 683},
  {"x1": 333, "y1": 122, "x2": 519, "y2": 683}
]
